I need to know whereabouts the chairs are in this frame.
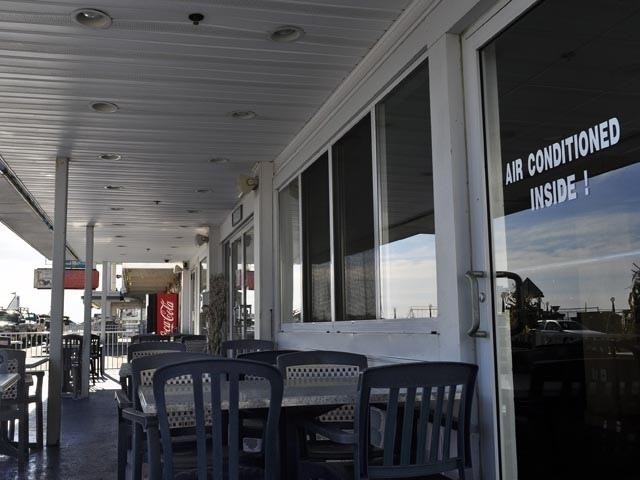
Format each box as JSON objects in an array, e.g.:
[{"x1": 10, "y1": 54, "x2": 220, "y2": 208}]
[{"x1": 0, "y1": 334, "x2": 100, "y2": 480}]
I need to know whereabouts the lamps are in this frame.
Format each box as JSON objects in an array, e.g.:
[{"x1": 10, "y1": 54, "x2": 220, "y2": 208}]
[
  {"x1": 194, "y1": 233, "x2": 208, "y2": 248},
  {"x1": 173, "y1": 264, "x2": 184, "y2": 274},
  {"x1": 236, "y1": 173, "x2": 258, "y2": 194}
]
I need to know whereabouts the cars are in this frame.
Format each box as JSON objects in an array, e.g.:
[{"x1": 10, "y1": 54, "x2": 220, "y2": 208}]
[
  {"x1": 0, "y1": 308, "x2": 78, "y2": 347},
  {"x1": 524, "y1": 320, "x2": 608, "y2": 345}
]
